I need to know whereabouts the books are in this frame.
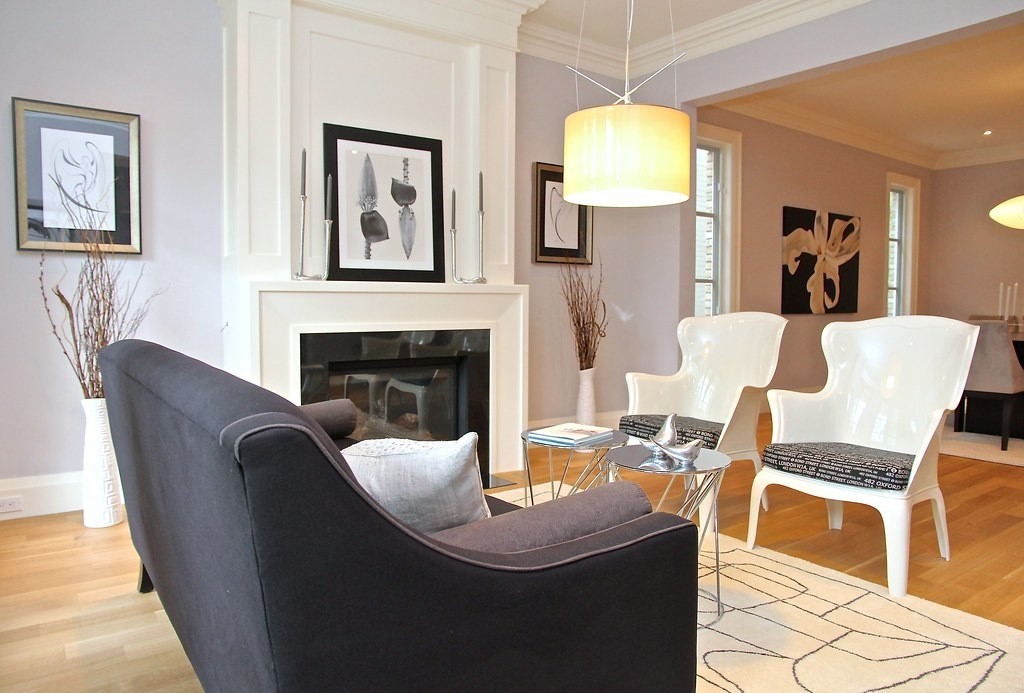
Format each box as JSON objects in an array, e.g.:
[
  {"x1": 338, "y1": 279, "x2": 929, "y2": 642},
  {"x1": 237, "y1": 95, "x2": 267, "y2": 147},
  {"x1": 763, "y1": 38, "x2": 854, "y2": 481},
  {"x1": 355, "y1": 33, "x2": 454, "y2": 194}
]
[{"x1": 528, "y1": 422, "x2": 613, "y2": 444}]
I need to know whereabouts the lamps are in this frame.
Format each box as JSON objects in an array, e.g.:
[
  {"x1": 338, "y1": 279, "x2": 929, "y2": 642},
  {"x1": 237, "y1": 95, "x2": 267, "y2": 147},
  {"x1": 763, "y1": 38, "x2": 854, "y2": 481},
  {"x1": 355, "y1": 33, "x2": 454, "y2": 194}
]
[
  {"x1": 988, "y1": 195, "x2": 1024, "y2": 230},
  {"x1": 564, "y1": 0, "x2": 692, "y2": 208}
]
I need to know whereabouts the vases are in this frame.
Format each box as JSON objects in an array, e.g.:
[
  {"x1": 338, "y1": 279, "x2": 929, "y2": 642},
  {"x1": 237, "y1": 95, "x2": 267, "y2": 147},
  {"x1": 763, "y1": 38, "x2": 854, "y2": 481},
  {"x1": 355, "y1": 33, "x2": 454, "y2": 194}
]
[
  {"x1": 81, "y1": 398, "x2": 125, "y2": 529},
  {"x1": 576, "y1": 367, "x2": 599, "y2": 429}
]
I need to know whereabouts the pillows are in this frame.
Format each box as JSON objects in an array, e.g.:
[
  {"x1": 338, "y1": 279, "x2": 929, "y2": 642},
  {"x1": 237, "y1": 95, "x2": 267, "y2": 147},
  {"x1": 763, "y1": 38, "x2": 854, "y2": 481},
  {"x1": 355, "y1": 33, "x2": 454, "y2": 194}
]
[{"x1": 340, "y1": 433, "x2": 492, "y2": 533}]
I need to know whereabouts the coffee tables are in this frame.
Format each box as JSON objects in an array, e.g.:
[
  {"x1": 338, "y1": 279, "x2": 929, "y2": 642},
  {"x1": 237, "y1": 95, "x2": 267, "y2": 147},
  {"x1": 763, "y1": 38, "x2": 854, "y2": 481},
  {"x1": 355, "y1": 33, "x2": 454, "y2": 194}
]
[
  {"x1": 605, "y1": 446, "x2": 732, "y2": 637},
  {"x1": 520, "y1": 422, "x2": 629, "y2": 506}
]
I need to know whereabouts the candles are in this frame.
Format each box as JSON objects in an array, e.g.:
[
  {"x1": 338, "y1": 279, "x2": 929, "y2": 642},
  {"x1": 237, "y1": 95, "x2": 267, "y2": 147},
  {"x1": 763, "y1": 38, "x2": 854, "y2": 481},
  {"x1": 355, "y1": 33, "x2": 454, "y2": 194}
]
[
  {"x1": 451, "y1": 188, "x2": 457, "y2": 228},
  {"x1": 1004, "y1": 287, "x2": 1012, "y2": 322},
  {"x1": 300, "y1": 148, "x2": 307, "y2": 197},
  {"x1": 999, "y1": 282, "x2": 1004, "y2": 317},
  {"x1": 478, "y1": 172, "x2": 483, "y2": 211},
  {"x1": 1013, "y1": 282, "x2": 1018, "y2": 315},
  {"x1": 327, "y1": 172, "x2": 333, "y2": 220}
]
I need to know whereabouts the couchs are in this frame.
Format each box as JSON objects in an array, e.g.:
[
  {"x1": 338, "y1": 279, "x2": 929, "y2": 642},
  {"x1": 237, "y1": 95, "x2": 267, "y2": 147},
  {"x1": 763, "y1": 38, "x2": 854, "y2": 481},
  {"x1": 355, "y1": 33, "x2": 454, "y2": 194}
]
[{"x1": 97, "y1": 339, "x2": 698, "y2": 693}]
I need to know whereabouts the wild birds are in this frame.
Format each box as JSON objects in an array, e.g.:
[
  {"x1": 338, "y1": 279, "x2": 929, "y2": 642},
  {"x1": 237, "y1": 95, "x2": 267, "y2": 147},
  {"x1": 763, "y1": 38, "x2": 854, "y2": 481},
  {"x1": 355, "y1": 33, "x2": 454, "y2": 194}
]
[
  {"x1": 640, "y1": 414, "x2": 677, "y2": 452},
  {"x1": 638, "y1": 457, "x2": 674, "y2": 471},
  {"x1": 649, "y1": 436, "x2": 703, "y2": 466}
]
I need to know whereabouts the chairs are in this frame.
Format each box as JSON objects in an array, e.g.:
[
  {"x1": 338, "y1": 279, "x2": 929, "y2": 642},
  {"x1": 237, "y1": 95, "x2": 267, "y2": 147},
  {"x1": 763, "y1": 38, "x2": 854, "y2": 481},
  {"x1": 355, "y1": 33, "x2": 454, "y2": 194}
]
[
  {"x1": 626, "y1": 311, "x2": 788, "y2": 536},
  {"x1": 962, "y1": 319, "x2": 1024, "y2": 450},
  {"x1": 747, "y1": 314, "x2": 981, "y2": 597}
]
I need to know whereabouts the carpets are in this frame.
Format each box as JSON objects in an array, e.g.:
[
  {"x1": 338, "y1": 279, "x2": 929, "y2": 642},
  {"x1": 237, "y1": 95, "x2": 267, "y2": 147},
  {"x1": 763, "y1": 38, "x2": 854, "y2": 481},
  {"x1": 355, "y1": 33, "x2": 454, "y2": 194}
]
[
  {"x1": 938, "y1": 423, "x2": 1024, "y2": 468},
  {"x1": 488, "y1": 479, "x2": 1024, "y2": 693}
]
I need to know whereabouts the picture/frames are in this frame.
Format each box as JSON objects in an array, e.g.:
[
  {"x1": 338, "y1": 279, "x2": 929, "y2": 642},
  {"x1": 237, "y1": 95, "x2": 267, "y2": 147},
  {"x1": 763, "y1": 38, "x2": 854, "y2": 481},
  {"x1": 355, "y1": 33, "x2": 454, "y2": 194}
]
[
  {"x1": 12, "y1": 97, "x2": 142, "y2": 256},
  {"x1": 534, "y1": 161, "x2": 594, "y2": 267},
  {"x1": 323, "y1": 122, "x2": 446, "y2": 283}
]
